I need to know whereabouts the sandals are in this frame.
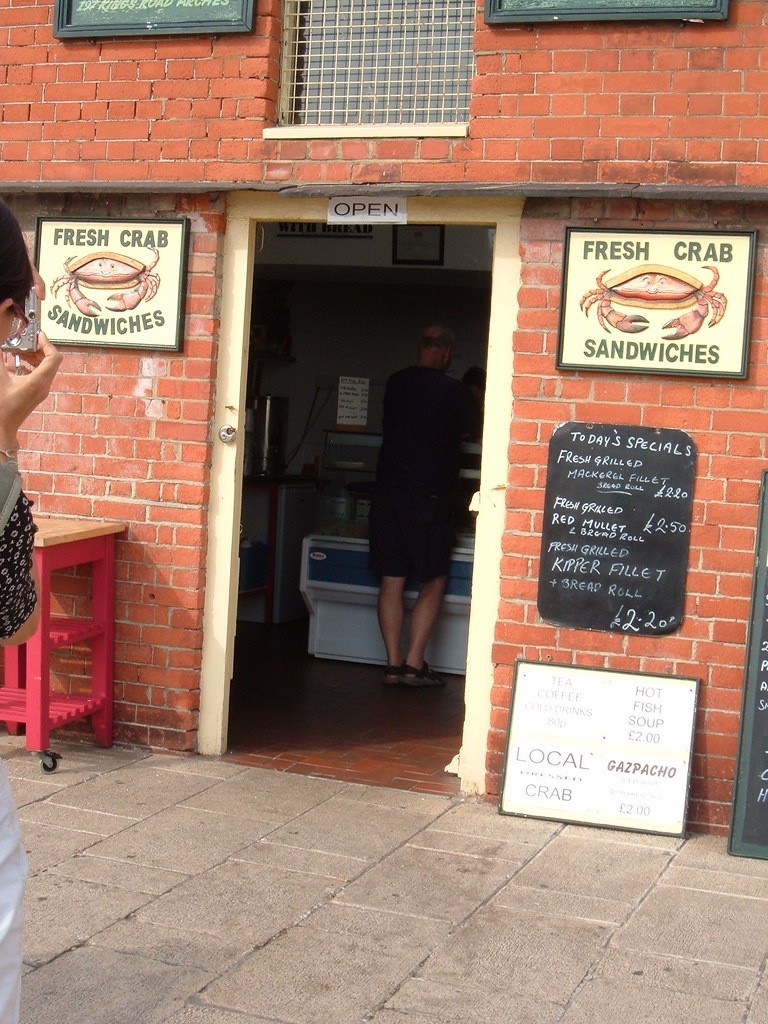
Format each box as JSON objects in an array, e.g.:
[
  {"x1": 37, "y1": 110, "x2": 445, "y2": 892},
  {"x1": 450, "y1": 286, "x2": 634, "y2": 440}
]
[
  {"x1": 382, "y1": 665, "x2": 402, "y2": 683},
  {"x1": 398, "y1": 659, "x2": 447, "y2": 686}
]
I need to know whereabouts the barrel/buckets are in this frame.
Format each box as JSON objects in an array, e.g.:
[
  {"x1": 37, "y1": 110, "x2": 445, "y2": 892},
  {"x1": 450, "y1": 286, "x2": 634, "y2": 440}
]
[{"x1": 249, "y1": 394, "x2": 288, "y2": 476}]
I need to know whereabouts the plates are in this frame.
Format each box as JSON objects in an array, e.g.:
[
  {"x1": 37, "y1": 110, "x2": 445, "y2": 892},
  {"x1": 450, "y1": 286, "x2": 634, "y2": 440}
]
[{"x1": 333, "y1": 460, "x2": 365, "y2": 470}]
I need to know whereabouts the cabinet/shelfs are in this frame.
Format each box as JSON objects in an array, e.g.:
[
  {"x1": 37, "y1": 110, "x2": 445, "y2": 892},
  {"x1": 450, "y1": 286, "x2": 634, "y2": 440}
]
[
  {"x1": 238, "y1": 474, "x2": 314, "y2": 625},
  {"x1": 0, "y1": 517, "x2": 125, "y2": 775},
  {"x1": 301, "y1": 428, "x2": 481, "y2": 676}
]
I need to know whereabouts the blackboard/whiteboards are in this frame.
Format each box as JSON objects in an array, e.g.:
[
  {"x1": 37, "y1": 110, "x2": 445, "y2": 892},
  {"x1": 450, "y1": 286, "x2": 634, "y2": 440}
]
[
  {"x1": 709, "y1": 463, "x2": 768, "y2": 861},
  {"x1": 538, "y1": 420, "x2": 696, "y2": 637},
  {"x1": 498, "y1": 656, "x2": 701, "y2": 840}
]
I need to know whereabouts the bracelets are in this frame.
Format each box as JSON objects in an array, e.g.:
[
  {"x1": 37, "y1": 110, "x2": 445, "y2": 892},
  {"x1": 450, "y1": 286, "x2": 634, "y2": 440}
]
[{"x1": 0, "y1": 443, "x2": 19, "y2": 459}]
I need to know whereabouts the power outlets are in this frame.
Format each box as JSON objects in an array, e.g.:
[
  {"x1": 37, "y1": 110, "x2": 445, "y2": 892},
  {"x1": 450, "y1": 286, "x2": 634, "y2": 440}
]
[{"x1": 315, "y1": 374, "x2": 341, "y2": 392}]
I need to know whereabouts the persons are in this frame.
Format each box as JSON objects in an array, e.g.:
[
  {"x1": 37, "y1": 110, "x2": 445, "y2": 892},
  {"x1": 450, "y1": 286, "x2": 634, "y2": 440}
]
[
  {"x1": 463, "y1": 365, "x2": 486, "y2": 396},
  {"x1": 0, "y1": 202, "x2": 64, "y2": 1024},
  {"x1": 365, "y1": 326, "x2": 482, "y2": 686}
]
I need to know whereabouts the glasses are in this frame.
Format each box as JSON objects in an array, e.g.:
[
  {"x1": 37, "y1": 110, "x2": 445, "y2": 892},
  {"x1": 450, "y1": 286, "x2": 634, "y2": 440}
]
[{"x1": 8, "y1": 303, "x2": 30, "y2": 340}]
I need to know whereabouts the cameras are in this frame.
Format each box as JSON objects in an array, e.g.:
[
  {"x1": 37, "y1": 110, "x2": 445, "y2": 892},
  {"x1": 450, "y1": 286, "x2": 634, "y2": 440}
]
[{"x1": 1, "y1": 286, "x2": 41, "y2": 352}]
